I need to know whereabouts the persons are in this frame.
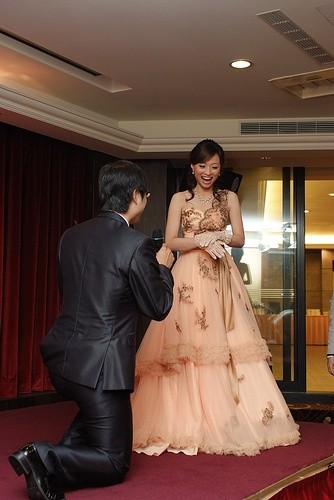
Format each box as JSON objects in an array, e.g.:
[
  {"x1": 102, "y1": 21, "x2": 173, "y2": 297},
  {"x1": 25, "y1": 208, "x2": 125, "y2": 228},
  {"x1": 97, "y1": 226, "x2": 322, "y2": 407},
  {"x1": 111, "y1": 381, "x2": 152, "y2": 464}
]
[
  {"x1": 327, "y1": 294, "x2": 334, "y2": 375},
  {"x1": 163, "y1": 139, "x2": 245, "y2": 457},
  {"x1": 231, "y1": 247, "x2": 252, "y2": 285},
  {"x1": 8, "y1": 160, "x2": 175, "y2": 500}
]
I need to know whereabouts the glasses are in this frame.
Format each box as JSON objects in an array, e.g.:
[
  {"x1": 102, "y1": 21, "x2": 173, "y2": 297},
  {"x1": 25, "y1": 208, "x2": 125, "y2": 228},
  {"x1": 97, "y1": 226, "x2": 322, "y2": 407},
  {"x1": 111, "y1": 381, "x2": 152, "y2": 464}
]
[{"x1": 136, "y1": 189, "x2": 151, "y2": 198}]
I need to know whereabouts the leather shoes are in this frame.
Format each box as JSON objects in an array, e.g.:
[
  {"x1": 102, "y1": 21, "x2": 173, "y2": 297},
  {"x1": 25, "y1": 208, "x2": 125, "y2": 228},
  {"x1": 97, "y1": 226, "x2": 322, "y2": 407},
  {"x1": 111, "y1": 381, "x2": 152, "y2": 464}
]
[{"x1": 8, "y1": 442, "x2": 65, "y2": 500}]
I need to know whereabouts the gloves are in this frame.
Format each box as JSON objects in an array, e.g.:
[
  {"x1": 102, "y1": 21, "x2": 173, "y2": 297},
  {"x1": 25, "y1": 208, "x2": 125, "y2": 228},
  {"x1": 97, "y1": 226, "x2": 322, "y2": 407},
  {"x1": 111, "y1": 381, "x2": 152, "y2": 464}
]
[{"x1": 195, "y1": 228, "x2": 232, "y2": 260}]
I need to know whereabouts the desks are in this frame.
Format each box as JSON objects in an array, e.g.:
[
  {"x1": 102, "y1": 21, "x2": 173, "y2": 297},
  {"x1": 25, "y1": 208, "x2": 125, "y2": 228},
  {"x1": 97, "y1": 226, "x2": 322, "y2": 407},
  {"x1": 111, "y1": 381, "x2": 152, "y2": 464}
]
[
  {"x1": 274, "y1": 315, "x2": 328, "y2": 345},
  {"x1": 255, "y1": 314, "x2": 274, "y2": 339}
]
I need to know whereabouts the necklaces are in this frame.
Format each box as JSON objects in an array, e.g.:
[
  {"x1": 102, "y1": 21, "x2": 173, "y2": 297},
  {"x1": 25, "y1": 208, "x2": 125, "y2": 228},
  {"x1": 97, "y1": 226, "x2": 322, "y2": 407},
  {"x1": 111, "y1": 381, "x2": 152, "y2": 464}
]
[{"x1": 193, "y1": 188, "x2": 214, "y2": 205}]
